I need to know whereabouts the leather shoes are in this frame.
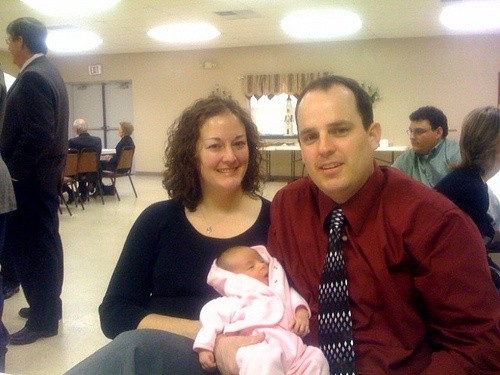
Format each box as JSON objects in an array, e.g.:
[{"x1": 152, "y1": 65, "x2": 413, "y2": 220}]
[
  {"x1": 19, "y1": 308, "x2": 62, "y2": 320},
  {"x1": 8, "y1": 323, "x2": 58, "y2": 345}
]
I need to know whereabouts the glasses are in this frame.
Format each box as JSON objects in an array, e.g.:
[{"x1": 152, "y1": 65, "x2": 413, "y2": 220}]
[
  {"x1": 406, "y1": 127, "x2": 433, "y2": 136},
  {"x1": 4, "y1": 37, "x2": 12, "y2": 45}
]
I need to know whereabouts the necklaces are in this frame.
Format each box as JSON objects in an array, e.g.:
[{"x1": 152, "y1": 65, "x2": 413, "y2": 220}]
[{"x1": 198, "y1": 205, "x2": 236, "y2": 232}]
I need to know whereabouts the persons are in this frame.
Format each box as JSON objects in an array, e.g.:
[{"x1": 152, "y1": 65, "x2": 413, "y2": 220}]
[
  {"x1": 63, "y1": 94, "x2": 273, "y2": 375},
  {"x1": 193, "y1": 244, "x2": 330, "y2": 375},
  {"x1": 265, "y1": 75, "x2": 500, "y2": 375},
  {"x1": 0, "y1": 16, "x2": 69, "y2": 375},
  {"x1": 389, "y1": 105, "x2": 462, "y2": 190},
  {"x1": 63, "y1": 118, "x2": 102, "y2": 205},
  {"x1": 431, "y1": 106, "x2": 500, "y2": 290},
  {"x1": 100, "y1": 122, "x2": 135, "y2": 196}
]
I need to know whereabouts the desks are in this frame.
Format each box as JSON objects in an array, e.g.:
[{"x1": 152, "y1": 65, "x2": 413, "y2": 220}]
[{"x1": 257, "y1": 143, "x2": 407, "y2": 187}]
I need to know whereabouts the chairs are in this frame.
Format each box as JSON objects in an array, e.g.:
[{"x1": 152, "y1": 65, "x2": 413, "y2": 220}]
[{"x1": 57, "y1": 147, "x2": 138, "y2": 216}]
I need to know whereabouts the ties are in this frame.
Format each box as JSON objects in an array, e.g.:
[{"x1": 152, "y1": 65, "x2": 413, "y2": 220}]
[{"x1": 318, "y1": 208, "x2": 356, "y2": 375}]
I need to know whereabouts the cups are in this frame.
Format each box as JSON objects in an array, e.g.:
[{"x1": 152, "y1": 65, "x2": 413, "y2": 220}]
[{"x1": 379, "y1": 139, "x2": 389, "y2": 149}]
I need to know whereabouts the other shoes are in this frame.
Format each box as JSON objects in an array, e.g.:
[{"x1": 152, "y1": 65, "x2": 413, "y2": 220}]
[
  {"x1": 3, "y1": 285, "x2": 19, "y2": 300},
  {"x1": 67, "y1": 192, "x2": 78, "y2": 204},
  {"x1": 78, "y1": 193, "x2": 87, "y2": 203}
]
